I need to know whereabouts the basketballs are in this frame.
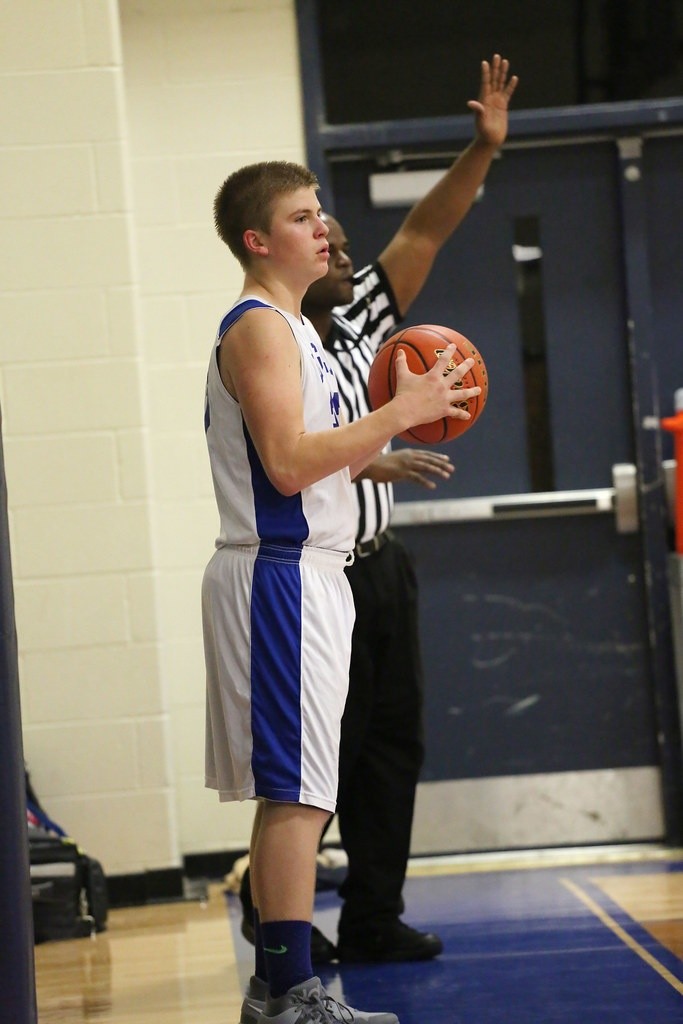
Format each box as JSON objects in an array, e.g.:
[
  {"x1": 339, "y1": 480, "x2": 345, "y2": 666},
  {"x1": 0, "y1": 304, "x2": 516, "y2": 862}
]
[{"x1": 366, "y1": 322, "x2": 490, "y2": 447}]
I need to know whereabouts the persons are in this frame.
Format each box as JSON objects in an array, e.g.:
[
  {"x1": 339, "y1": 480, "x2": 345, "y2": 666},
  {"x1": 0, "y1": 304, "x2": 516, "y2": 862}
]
[
  {"x1": 238, "y1": 54, "x2": 517, "y2": 964},
  {"x1": 201, "y1": 161, "x2": 482, "y2": 1024}
]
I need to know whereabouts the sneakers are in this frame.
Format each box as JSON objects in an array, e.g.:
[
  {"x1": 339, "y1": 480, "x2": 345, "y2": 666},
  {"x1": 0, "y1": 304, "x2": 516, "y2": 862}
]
[
  {"x1": 257, "y1": 976, "x2": 401, "y2": 1024},
  {"x1": 239, "y1": 976, "x2": 264, "y2": 1024}
]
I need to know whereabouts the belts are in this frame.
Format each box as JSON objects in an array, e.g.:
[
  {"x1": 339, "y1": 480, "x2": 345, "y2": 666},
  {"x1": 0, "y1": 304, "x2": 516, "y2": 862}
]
[{"x1": 347, "y1": 529, "x2": 394, "y2": 564}]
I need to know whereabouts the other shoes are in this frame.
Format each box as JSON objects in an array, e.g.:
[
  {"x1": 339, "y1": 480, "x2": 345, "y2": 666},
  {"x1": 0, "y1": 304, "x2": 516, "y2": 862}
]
[
  {"x1": 336, "y1": 911, "x2": 443, "y2": 961},
  {"x1": 240, "y1": 909, "x2": 347, "y2": 970}
]
